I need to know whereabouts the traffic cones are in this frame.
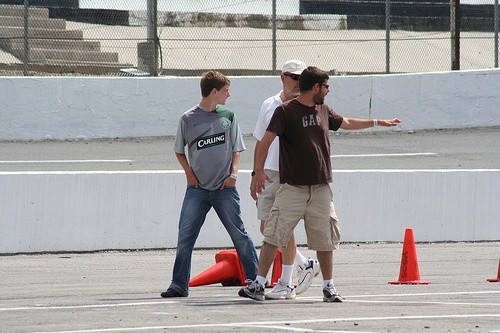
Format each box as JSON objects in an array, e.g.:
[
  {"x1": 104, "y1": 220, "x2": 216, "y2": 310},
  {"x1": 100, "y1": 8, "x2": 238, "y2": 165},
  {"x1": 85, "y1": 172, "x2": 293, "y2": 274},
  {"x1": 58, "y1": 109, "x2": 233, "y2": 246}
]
[
  {"x1": 265, "y1": 248, "x2": 298, "y2": 288},
  {"x1": 388, "y1": 228, "x2": 432, "y2": 285},
  {"x1": 189, "y1": 250, "x2": 247, "y2": 286}
]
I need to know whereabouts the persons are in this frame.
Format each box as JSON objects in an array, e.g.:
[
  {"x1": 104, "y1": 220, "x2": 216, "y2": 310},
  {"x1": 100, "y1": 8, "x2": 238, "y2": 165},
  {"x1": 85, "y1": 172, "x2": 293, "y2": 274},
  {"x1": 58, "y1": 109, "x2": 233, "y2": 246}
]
[
  {"x1": 160, "y1": 71, "x2": 259, "y2": 298},
  {"x1": 238, "y1": 65, "x2": 402, "y2": 302},
  {"x1": 250, "y1": 61, "x2": 322, "y2": 299}
]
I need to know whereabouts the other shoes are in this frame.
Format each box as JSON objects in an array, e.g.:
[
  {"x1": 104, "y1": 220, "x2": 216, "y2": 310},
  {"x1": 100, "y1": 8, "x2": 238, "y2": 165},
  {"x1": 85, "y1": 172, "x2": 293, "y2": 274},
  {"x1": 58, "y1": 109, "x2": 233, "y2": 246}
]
[{"x1": 161, "y1": 289, "x2": 187, "y2": 297}]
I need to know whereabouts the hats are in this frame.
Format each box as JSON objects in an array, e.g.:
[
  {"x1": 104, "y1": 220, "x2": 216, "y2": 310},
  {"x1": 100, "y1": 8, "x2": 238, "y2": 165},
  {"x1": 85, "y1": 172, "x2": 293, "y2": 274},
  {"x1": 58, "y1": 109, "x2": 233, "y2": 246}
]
[{"x1": 281, "y1": 59, "x2": 307, "y2": 75}]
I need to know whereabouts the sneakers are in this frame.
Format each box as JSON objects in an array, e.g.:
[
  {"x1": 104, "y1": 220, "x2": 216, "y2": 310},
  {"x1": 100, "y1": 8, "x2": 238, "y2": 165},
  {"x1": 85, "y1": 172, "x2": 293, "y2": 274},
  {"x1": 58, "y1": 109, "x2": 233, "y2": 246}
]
[
  {"x1": 295, "y1": 257, "x2": 321, "y2": 295},
  {"x1": 238, "y1": 279, "x2": 265, "y2": 301},
  {"x1": 322, "y1": 286, "x2": 344, "y2": 302},
  {"x1": 264, "y1": 279, "x2": 296, "y2": 299}
]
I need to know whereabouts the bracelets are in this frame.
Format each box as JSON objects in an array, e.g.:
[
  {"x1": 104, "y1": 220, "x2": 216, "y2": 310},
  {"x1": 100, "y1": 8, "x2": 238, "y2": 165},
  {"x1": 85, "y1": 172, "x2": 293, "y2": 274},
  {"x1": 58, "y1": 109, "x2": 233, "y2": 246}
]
[
  {"x1": 230, "y1": 173, "x2": 237, "y2": 181},
  {"x1": 251, "y1": 171, "x2": 255, "y2": 176},
  {"x1": 372, "y1": 118, "x2": 378, "y2": 127}
]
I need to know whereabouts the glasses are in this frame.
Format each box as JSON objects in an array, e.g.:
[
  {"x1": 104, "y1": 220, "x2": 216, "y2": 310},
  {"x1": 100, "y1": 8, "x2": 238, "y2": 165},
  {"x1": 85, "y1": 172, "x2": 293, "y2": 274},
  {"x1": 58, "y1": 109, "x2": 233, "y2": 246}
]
[
  {"x1": 314, "y1": 82, "x2": 329, "y2": 89},
  {"x1": 283, "y1": 73, "x2": 300, "y2": 82}
]
